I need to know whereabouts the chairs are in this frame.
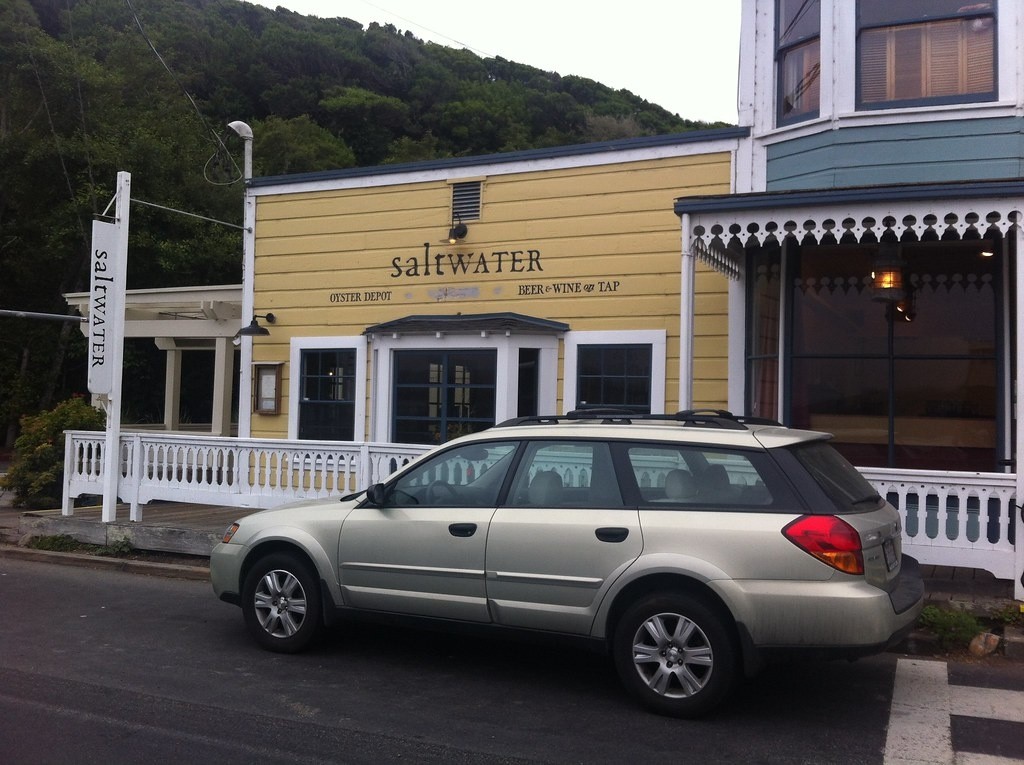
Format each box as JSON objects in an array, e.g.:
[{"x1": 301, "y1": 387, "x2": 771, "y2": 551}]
[{"x1": 525, "y1": 470, "x2": 563, "y2": 507}]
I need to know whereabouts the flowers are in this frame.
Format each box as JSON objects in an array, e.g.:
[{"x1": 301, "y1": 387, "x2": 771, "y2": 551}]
[{"x1": 433, "y1": 423, "x2": 472, "y2": 441}]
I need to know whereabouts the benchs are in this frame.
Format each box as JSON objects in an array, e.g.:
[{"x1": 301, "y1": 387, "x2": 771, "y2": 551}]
[{"x1": 648, "y1": 463, "x2": 732, "y2": 503}]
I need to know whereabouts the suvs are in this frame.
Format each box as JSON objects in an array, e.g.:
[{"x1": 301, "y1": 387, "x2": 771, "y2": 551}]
[{"x1": 209, "y1": 408, "x2": 923, "y2": 723}]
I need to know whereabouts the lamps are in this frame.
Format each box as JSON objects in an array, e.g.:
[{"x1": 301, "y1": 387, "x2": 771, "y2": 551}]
[
  {"x1": 237, "y1": 313, "x2": 276, "y2": 337},
  {"x1": 447, "y1": 212, "x2": 467, "y2": 245},
  {"x1": 869, "y1": 241, "x2": 908, "y2": 303}
]
